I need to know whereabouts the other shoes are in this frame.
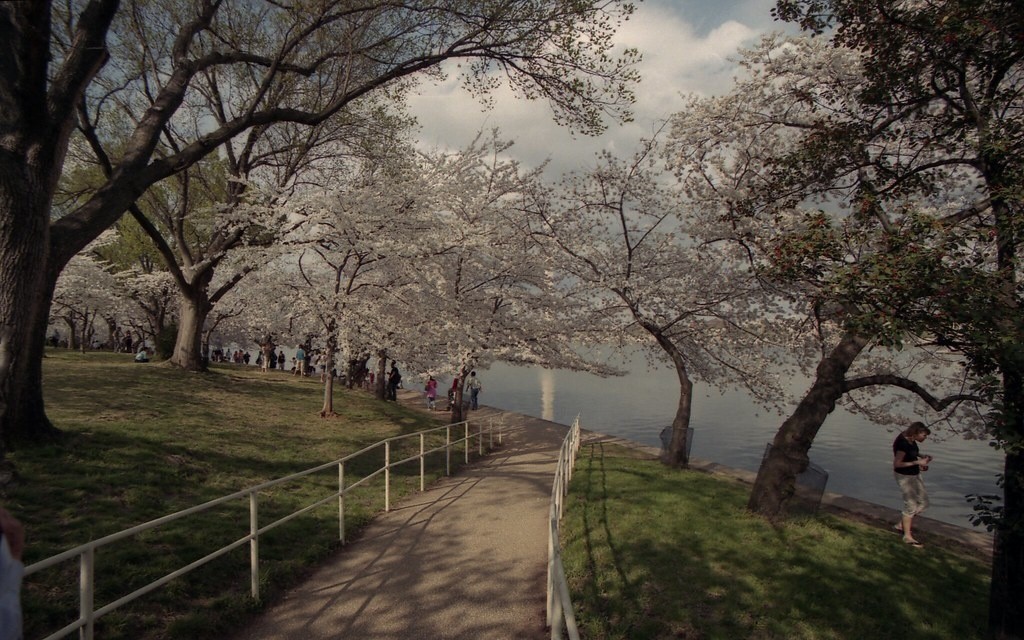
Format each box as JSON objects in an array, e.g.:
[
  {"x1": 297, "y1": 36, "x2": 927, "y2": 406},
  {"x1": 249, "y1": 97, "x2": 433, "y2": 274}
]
[{"x1": 428, "y1": 406, "x2": 436, "y2": 411}]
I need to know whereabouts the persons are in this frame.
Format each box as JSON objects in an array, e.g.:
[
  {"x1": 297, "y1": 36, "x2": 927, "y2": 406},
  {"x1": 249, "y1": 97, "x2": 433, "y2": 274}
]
[
  {"x1": 451, "y1": 373, "x2": 461, "y2": 405},
  {"x1": 893, "y1": 421, "x2": 933, "y2": 548},
  {"x1": 386, "y1": 364, "x2": 400, "y2": 402},
  {"x1": 46, "y1": 324, "x2": 172, "y2": 363},
  {"x1": 209, "y1": 341, "x2": 376, "y2": 392},
  {"x1": 383, "y1": 360, "x2": 398, "y2": 389},
  {"x1": 424, "y1": 375, "x2": 437, "y2": 411},
  {"x1": 466, "y1": 372, "x2": 482, "y2": 410}
]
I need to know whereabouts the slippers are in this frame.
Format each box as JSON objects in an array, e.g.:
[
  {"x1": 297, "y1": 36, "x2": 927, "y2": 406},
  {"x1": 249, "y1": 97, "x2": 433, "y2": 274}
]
[{"x1": 903, "y1": 537, "x2": 925, "y2": 549}]
